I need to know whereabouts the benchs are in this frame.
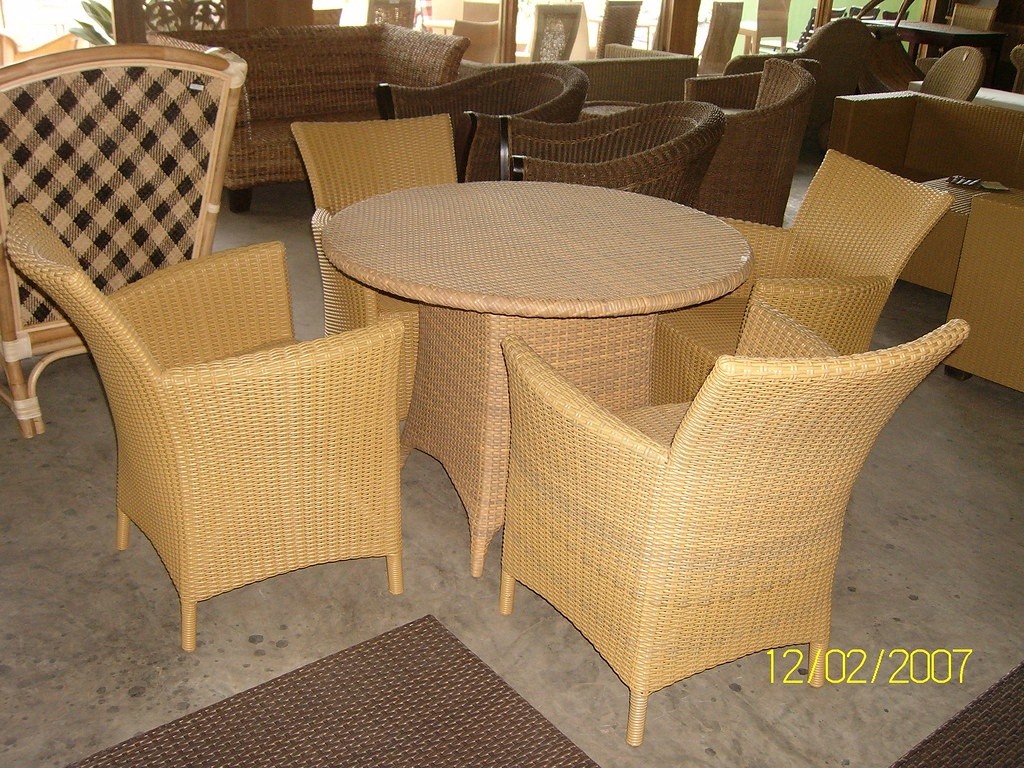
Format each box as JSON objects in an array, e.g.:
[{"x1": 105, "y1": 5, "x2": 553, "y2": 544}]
[
  {"x1": 457, "y1": 43, "x2": 700, "y2": 106},
  {"x1": 142, "y1": 23, "x2": 471, "y2": 214}
]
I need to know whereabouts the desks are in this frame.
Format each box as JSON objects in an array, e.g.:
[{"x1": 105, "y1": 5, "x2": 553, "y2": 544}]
[
  {"x1": 909, "y1": 81, "x2": 1024, "y2": 113},
  {"x1": 860, "y1": 18, "x2": 1007, "y2": 66},
  {"x1": 422, "y1": 20, "x2": 456, "y2": 34},
  {"x1": 322, "y1": 176, "x2": 754, "y2": 577}
]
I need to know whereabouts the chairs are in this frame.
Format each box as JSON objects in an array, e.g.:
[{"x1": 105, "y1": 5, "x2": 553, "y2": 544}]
[{"x1": 0, "y1": 0, "x2": 1024, "y2": 747}]
